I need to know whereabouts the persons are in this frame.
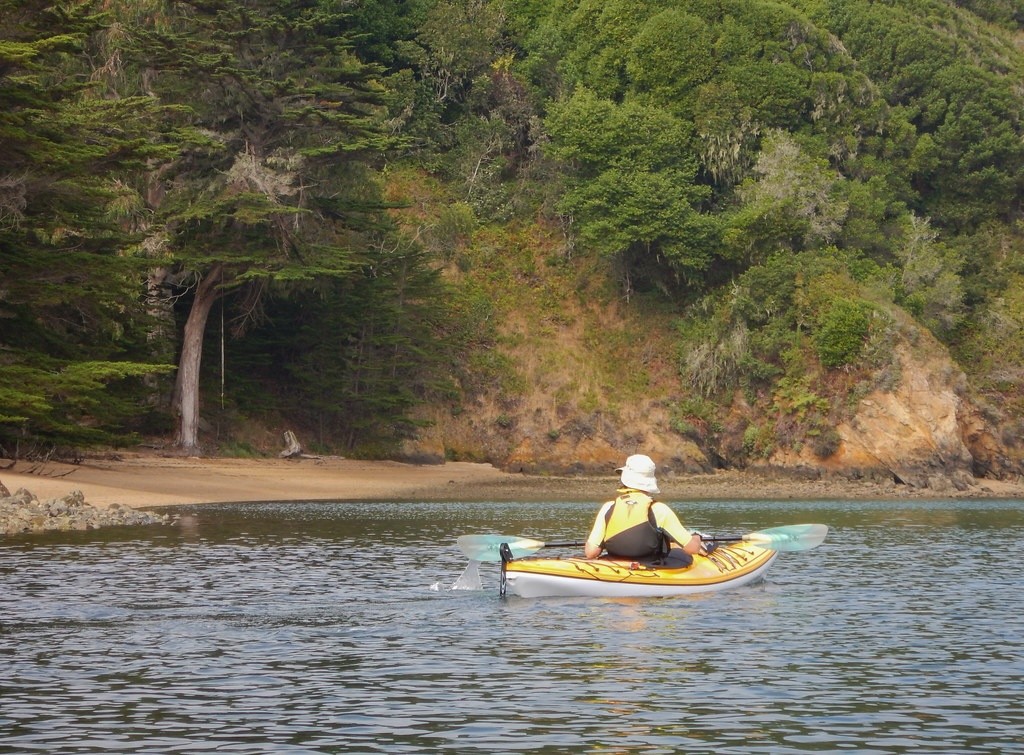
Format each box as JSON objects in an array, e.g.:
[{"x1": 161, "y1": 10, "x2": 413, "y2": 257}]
[{"x1": 584, "y1": 454, "x2": 703, "y2": 570}]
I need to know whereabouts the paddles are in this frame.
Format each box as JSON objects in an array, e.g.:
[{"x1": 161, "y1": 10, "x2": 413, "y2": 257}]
[{"x1": 455, "y1": 522, "x2": 830, "y2": 563}]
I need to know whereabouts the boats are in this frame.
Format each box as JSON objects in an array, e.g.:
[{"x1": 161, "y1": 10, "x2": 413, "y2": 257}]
[{"x1": 499, "y1": 540, "x2": 781, "y2": 601}]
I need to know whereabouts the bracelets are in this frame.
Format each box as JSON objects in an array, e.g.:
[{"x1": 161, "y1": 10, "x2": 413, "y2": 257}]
[{"x1": 692, "y1": 532, "x2": 702, "y2": 537}]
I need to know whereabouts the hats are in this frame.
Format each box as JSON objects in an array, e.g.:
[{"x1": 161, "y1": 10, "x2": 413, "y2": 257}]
[{"x1": 615, "y1": 454, "x2": 660, "y2": 493}]
